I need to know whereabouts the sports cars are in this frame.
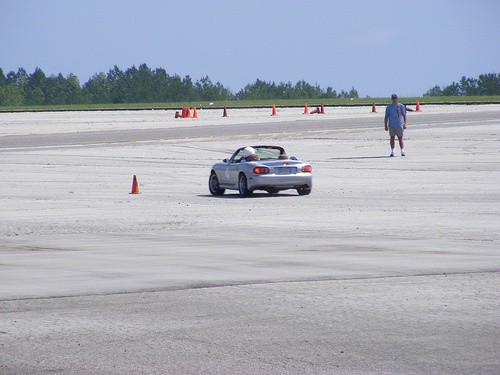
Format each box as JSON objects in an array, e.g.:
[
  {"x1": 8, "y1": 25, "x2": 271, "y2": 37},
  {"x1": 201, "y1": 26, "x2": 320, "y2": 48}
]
[{"x1": 208, "y1": 145, "x2": 313, "y2": 198}]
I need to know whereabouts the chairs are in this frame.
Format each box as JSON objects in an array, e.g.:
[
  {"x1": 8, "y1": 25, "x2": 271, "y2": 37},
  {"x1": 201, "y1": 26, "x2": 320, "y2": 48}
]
[
  {"x1": 278, "y1": 154, "x2": 288, "y2": 160},
  {"x1": 248, "y1": 155, "x2": 261, "y2": 162}
]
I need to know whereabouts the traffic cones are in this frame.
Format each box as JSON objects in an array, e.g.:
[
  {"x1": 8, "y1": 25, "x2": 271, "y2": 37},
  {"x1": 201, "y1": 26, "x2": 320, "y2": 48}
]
[
  {"x1": 271, "y1": 105, "x2": 278, "y2": 116},
  {"x1": 191, "y1": 107, "x2": 198, "y2": 118},
  {"x1": 175, "y1": 106, "x2": 193, "y2": 118},
  {"x1": 371, "y1": 102, "x2": 377, "y2": 113},
  {"x1": 129, "y1": 174, "x2": 142, "y2": 195},
  {"x1": 319, "y1": 104, "x2": 325, "y2": 115},
  {"x1": 302, "y1": 103, "x2": 310, "y2": 114},
  {"x1": 309, "y1": 107, "x2": 321, "y2": 114},
  {"x1": 414, "y1": 100, "x2": 423, "y2": 112},
  {"x1": 403, "y1": 105, "x2": 413, "y2": 112},
  {"x1": 221, "y1": 106, "x2": 228, "y2": 117}
]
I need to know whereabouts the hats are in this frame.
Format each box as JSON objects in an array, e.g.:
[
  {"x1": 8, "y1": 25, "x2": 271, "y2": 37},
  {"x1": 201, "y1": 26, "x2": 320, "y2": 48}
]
[
  {"x1": 392, "y1": 94, "x2": 398, "y2": 99},
  {"x1": 243, "y1": 147, "x2": 255, "y2": 158}
]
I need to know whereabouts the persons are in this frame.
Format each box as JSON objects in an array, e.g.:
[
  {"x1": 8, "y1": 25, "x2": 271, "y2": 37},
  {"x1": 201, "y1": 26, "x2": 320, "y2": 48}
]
[
  {"x1": 237, "y1": 147, "x2": 255, "y2": 162},
  {"x1": 385, "y1": 94, "x2": 406, "y2": 157}
]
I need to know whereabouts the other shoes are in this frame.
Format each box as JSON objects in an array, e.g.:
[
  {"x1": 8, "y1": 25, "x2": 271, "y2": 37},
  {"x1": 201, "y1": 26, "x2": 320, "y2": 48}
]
[
  {"x1": 390, "y1": 153, "x2": 394, "y2": 157},
  {"x1": 401, "y1": 152, "x2": 406, "y2": 156}
]
